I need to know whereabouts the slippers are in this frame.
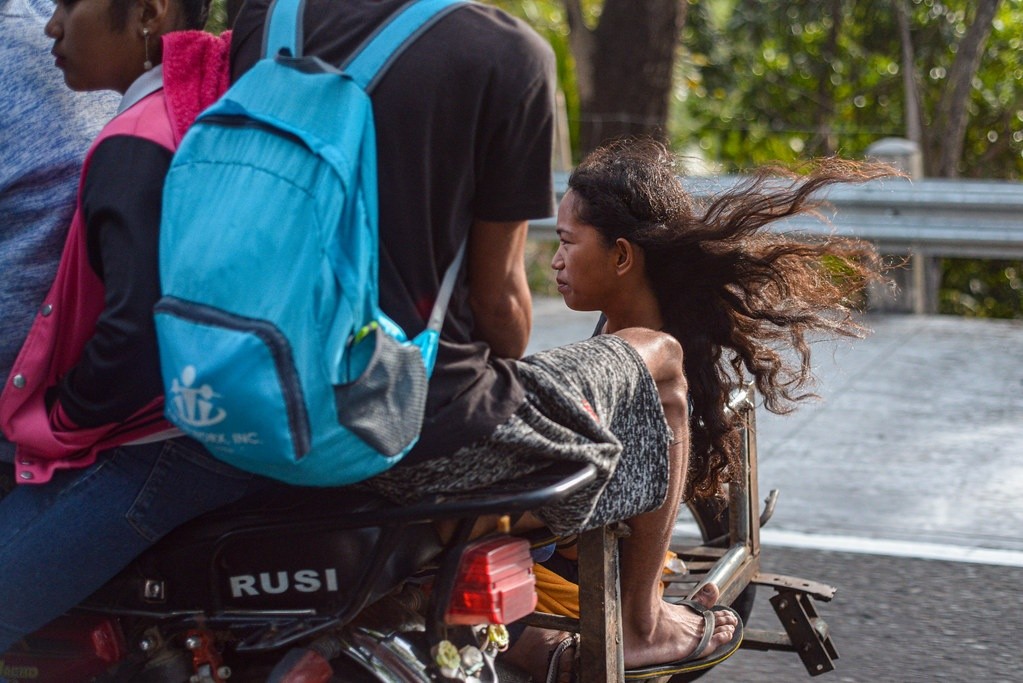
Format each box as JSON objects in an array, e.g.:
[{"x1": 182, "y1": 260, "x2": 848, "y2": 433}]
[{"x1": 623, "y1": 598, "x2": 745, "y2": 679}]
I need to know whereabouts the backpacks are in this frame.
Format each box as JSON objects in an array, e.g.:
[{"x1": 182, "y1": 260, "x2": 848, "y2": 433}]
[{"x1": 160, "y1": 0, "x2": 465, "y2": 486}]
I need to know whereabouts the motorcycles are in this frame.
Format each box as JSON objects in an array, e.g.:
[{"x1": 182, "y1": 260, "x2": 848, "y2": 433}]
[{"x1": 1, "y1": 386, "x2": 840, "y2": 683}]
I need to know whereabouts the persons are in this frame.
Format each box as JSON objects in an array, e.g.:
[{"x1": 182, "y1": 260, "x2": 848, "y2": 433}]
[
  {"x1": 492, "y1": 139, "x2": 903, "y2": 683},
  {"x1": 0, "y1": 0, "x2": 244, "y2": 655},
  {"x1": 0, "y1": 0, "x2": 124, "y2": 467},
  {"x1": 225, "y1": 1, "x2": 744, "y2": 679}
]
